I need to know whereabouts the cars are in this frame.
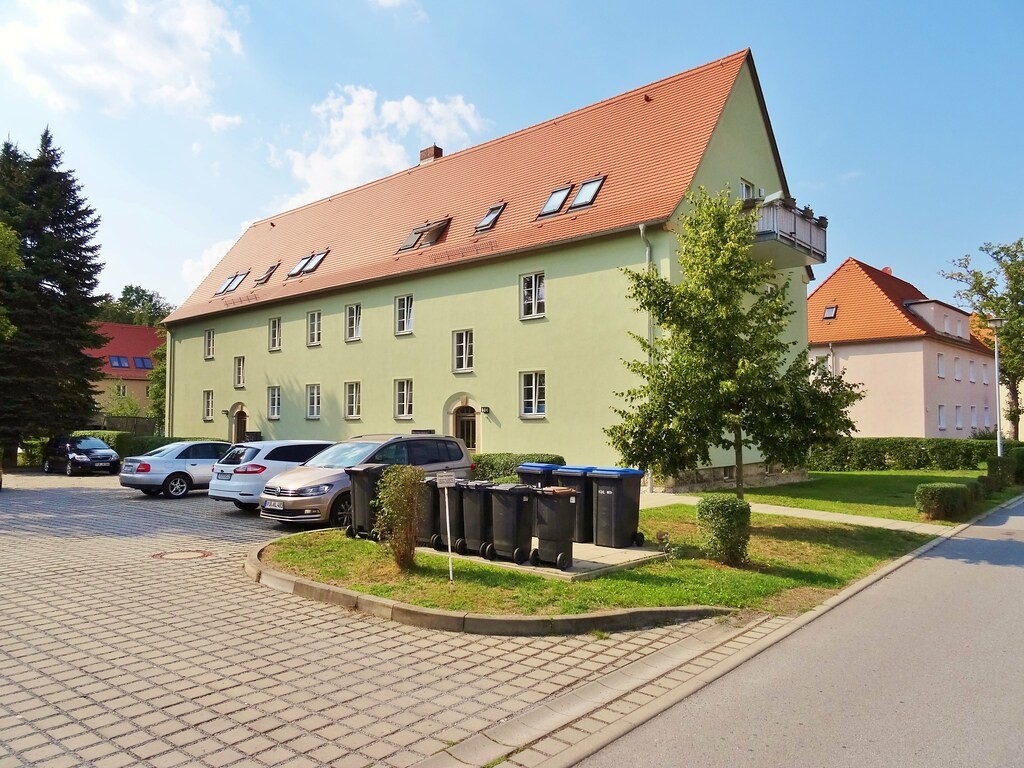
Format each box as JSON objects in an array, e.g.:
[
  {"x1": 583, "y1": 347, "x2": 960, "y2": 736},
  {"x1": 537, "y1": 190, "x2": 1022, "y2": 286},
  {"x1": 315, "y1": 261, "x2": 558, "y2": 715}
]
[
  {"x1": 119, "y1": 441, "x2": 243, "y2": 499},
  {"x1": 208, "y1": 440, "x2": 337, "y2": 511},
  {"x1": 41, "y1": 436, "x2": 120, "y2": 476}
]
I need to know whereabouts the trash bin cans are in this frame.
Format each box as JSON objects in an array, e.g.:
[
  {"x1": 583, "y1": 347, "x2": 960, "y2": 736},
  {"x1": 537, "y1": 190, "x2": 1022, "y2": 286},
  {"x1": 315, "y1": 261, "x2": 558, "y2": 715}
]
[
  {"x1": 515, "y1": 462, "x2": 562, "y2": 537},
  {"x1": 586, "y1": 467, "x2": 645, "y2": 549},
  {"x1": 551, "y1": 466, "x2": 597, "y2": 543},
  {"x1": 456, "y1": 479, "x2": 497, "y2": 558},
  {"x1": 432, "y1": 479, "x2": 469, "y2": 551},
  {"x1": 528, "y1": 486, "x2": 582, "y2": 569},
  {"x1": 410, "y1": 476, "x2": 440, "y2": 548},
  {"x1": 485, "y1": 482, "x2": 536, "y2": 565},
  {"x1": 344, "y1": 463, "x2": 392, "y2": 542}
]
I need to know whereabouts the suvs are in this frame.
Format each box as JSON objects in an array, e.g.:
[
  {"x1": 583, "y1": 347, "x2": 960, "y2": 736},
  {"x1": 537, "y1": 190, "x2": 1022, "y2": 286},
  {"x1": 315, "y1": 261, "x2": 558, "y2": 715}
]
[{"x1": 259, "y1": 434, "x2": 476, "y2": 528}]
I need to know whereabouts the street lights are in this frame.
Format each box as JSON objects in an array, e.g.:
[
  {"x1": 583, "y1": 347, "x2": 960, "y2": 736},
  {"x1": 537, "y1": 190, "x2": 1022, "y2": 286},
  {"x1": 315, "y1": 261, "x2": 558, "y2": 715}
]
[{"x1": 985, "y1": 318, "x2": 1006, "y2": 457}]
